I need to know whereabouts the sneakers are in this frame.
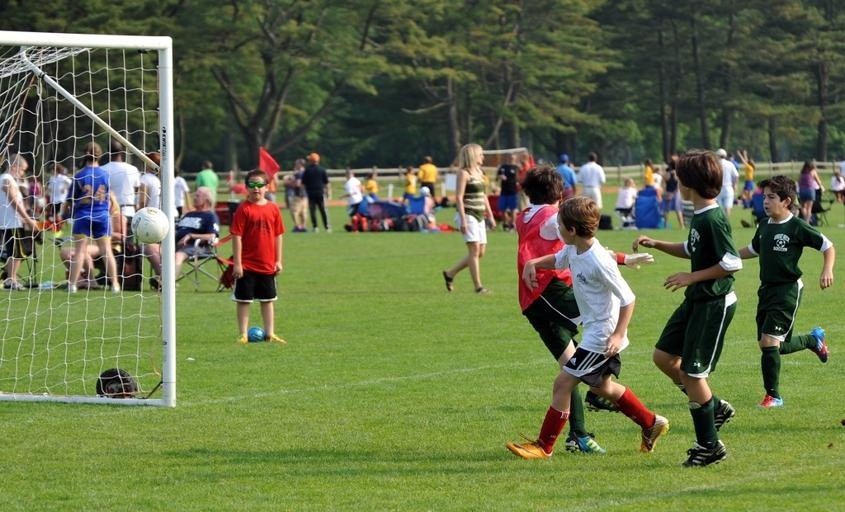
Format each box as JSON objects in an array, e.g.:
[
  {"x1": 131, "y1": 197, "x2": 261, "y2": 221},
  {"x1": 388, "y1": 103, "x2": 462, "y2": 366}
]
[
  {"x1": 291, "y1": 224, "x2": 331, "y2": 232},
  {"x1": 565, "y1": 431, "x2": 607, "y2": 455},
  {"x1": 236, "y1": 333, "x2": 249, "y2": 345},
  {"x1": 714, "y1": 399, "x2": 735, "y2": 431},
  {"x1": 505, "y1": 441, "x2": 554, "y2": 460},
  {"x1": 639, "y1": 412, "x2": 670, "y2": 453},
  {"x1": 584, "y1": 391, "x2": 622, "y2": 412},
  {"x1": 149, "y1": 278, "x2": 162, "y2": 291},
  {"x1": 760, "y1": 393, "x2": 784, "y2": 407},
  {"x1": 3, "y1": 279, "x2": 27, "y2": 290},
  {"x1": 682, "y1": 440, "x2": 726, "y2": 467},
  {"x1": 264, "y1": 334, "x2": 287, "y2": 344},
  {"x1": 809, "y1": 327, "x2": 829, "y2": 363},
  {"x1": 66, "y1": 277, "x2": 119, "y2": 294}
]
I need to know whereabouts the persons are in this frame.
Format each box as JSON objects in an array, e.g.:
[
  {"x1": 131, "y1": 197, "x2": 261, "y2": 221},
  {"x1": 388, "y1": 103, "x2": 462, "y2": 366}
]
[
  {"x1": 267, "y1": 154, "x2": 439, "y2": 232},
  {"x1": 737, "y1": 176, "x2": 836, "y2": 410},
  {"x1": 0, "y1": 136, "x2": 220, "y2": 294},
  {"x1": 632, "y1": 149, "x2": 744, "y2": 469},
  {"x1": 443, "y1": 143, "x2": 495, "y2": 296},
  {"x1": 230, "y1": 170, "x2": 286, "y2": 343},
  {"x1": 493, "y1": 146, "x2": 845, "y2": 236},
  {"x1": 506, "y1": 196, "x2": 669, "y2": 458},
  {"x1": 514, "y1": 163, "x2": 655, "y2": 454}
]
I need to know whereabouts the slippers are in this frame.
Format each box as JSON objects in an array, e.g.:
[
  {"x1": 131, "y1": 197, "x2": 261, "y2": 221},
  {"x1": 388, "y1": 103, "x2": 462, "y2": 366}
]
[
  {"x1": 443, "y1": 270, "x2": 453, "y2": 292},
  {"x1": 476, "y1": 287, "x2": 490, "y2": 293}
]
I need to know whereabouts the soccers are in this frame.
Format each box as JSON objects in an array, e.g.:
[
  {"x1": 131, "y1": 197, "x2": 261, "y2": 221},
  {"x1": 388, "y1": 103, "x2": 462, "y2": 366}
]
[
  {"x1": 131, "y1": 208, "x2": 169, "y2": 244},
  {"x1": 97, "y1": 368, "x2": 135, "y2": 400},
  {"x1": 249, "y1": 326, "x2": 265, "y2": 342}
]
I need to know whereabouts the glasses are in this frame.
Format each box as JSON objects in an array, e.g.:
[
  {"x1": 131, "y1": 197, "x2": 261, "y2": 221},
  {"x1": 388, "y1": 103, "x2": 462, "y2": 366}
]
[{"x1": 245, "y1": 181, "x2": 267, "y2": 188}]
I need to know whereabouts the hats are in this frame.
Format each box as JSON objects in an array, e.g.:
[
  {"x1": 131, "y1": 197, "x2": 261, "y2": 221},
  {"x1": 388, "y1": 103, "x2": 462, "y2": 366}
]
[{"x1": 306, "y1": 153, "x2": 320, "y2": 161}]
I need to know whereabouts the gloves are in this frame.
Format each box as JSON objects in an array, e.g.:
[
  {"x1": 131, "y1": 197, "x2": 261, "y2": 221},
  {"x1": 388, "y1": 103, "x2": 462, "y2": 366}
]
[{"x1": 617, "y1": 251, "x2": 655, "y2": 270}]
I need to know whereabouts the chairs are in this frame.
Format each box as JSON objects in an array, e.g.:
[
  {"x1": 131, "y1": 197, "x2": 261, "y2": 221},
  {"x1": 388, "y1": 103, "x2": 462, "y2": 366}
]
[
  {"x1": 817, "y1": 189, "x2": 833, "y2": 229},
  {"x1": 169, "y1": 235, "x2": 233, "y2": 292}
]
